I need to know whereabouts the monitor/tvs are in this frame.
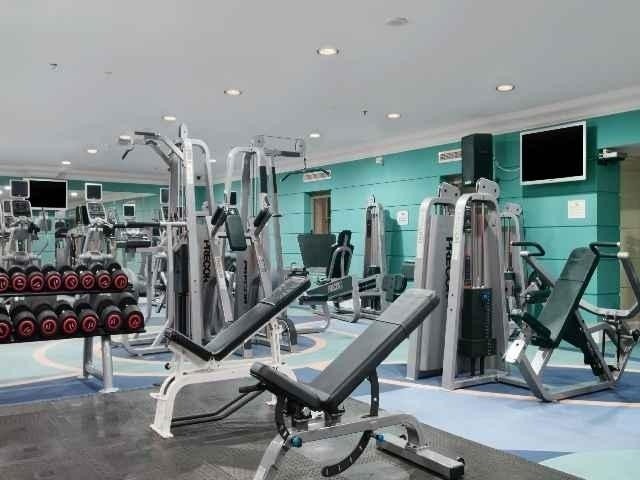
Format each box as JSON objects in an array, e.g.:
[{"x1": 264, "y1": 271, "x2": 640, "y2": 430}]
[
  {"x1": 123, "y1": 203, "x2": 136, "y2": 219},
  {"x1": 10, "y1": 179, "x2": 30, "y2": 198},
  {"x1": 27, "y1": 178, "x2": 68, "y2": 211},
  {"x1": 224, "y1": 191, "x2": 236, "y2": 205},
  {"x1": 160, "y1": 188, "x2": 168, "y2": 204},
  {"x1": 519, "y1": 120, "x2": 587, "y2": 185},
  {"x1": 85, "y1": 184, "x2": 102, "y2": 202}
]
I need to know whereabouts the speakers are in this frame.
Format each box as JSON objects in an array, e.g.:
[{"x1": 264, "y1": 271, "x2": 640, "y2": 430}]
[{"x1": 461, "y1": 133, "x2": 494, "y2": 186}]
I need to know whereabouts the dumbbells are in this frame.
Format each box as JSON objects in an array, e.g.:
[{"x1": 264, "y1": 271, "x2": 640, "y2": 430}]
[
  {"x1": 0, "y1": 261, "x2": 128, "y2": 294},
  {"x1": 0, "y1": 292, "x2": 144, "y2": 344}
]
